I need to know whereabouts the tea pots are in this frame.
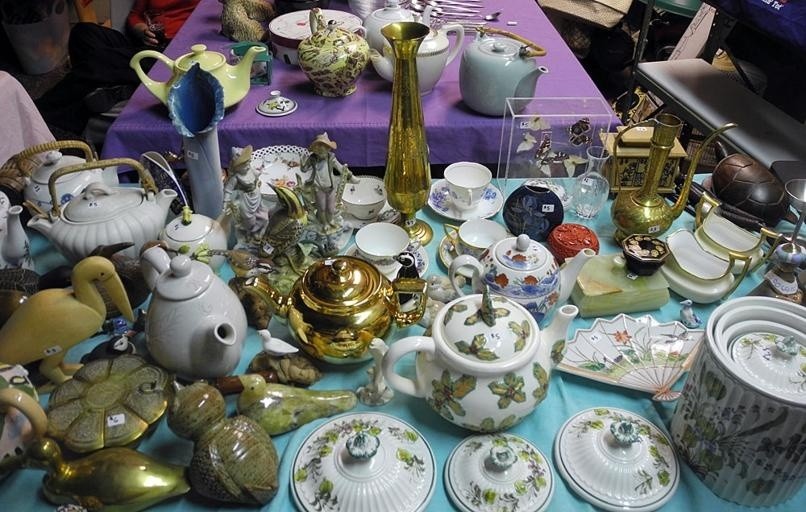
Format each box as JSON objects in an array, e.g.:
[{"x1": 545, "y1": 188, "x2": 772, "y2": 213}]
[
  {"x1": 611, "y1": 113, "x2": 740, "y2": 250},
  {"x1": 246, "y1": 259, "x2": 428, "y2": 368},
  {"x1": 2, "y1": 139, "x2": 121, "y2": 210},
  {"x1": 139, "y1": 246, "x2": 247, "y2": 385},
  {"x1": 383, "y1": 287, "x2": 580, "y2": 433},
  {"x1": 161, "y1": 207, "x2": 231, "y2": 271},
  {"x1": 26, "y1": 158, "x2": 178, "y2": 287},
  {"x1": 450, "y1": 235, "x2": 596, "y2": 323},
  {"x1": 129, "y1": 43, "x2": 265, "y2": 119},
  {"x1": 296, "y1": 0, "x2": 548, "y2": 117}
]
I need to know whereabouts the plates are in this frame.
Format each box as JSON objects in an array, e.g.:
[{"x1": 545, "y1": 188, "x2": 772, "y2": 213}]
[
  {"x1": 428, "y1": 177, "x2": 505, "y2": 223},
  {"x1": 438, "y1": 231, "x2": 481, "y2": 277},
  {"x1": 554, "y1": 311, "x2": 706, "y2": 406},
  {"x1": 341, "y1": 242, "x2": 430, "y2": 283},
  {"x1": 339, "y1": 174, "x2": 401, "y2": 230}
]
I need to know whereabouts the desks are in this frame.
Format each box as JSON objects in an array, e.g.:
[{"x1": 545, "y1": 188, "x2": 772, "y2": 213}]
[
  {"x1": 102, "y1": 0, "x2": 622, "y2": 175},
  {"x1": 0, "y1": 173, "x2": 806, "y2": 512}
]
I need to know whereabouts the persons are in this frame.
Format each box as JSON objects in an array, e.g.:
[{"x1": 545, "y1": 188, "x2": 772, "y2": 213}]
[
  {"x1": 299, "y1": 131, "x2": 360, "y2": 231},
  {"x1": 222, "y1": 144, "x2": 269, "y2": 244},
  {"x1": 67, "y1": 0, "x2": 201, "y2": 131}
]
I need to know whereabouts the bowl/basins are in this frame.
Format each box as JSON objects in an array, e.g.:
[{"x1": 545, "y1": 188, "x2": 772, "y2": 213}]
[{"x1": 267, "y1": 9, "x2": 365, "y2": 68}]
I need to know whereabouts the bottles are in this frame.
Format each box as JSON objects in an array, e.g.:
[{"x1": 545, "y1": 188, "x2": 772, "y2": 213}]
[{"x1": 571, "y1": 146, "x2": 611, "y2": 220}]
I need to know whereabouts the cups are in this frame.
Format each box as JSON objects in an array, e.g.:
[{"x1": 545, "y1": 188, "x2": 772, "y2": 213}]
[
  {"x1": 444, "y1": 162, "x2": 492, "y2": 212},
  {"x1": 355, "y1": 220, "x2": 423, "y2": 274},
  {"x1": 667, "y1": 297, "x2": 806, "y2": 508},
  {"x1": 693, "y1": 191, "x2": 783, "y2": 278},
  {"x1": 658, "y1": 226, "x2": 753, "y2": 305},
  {"x1": 341, "y1": 180, "x2": 388, "y2": 221},
  {"x1": 441, "y1": 218, "x2": 507, "y2": 257}
]
